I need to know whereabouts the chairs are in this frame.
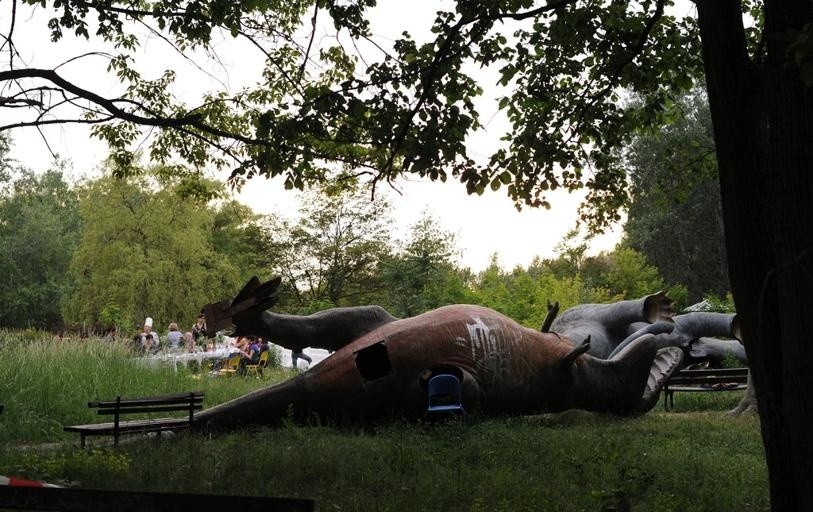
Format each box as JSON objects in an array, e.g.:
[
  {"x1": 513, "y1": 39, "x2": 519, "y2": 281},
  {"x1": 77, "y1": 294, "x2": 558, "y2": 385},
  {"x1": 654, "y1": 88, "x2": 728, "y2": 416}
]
[{"x1": 218, "y1": 350, "x2": 268, "y2": 377}]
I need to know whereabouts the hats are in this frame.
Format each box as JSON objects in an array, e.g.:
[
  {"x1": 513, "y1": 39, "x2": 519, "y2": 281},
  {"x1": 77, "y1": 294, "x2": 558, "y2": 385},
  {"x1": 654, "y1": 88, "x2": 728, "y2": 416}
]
[{"x1": 144, "y1": 317, "x2": 153, "y2": 327}]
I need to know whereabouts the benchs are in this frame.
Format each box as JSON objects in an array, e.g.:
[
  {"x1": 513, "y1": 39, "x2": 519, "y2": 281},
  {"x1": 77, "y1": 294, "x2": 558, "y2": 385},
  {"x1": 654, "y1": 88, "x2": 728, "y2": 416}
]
[
  {"x1": 664, "y1": 367, "x2": 747, "y2": 410},
  {"x1": 61, "y1": 391, "x2": 206, "y2": 447}
]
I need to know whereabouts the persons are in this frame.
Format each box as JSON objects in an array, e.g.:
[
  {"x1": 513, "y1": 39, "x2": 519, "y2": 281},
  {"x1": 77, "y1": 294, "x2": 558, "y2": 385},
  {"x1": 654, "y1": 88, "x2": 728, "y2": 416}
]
[
  {"x1": 291, "y1": 351, "x2": 312, "y2": 369},
  {"x1": 107, "y1": 312, "x2": 269, "y2": 380}
]
